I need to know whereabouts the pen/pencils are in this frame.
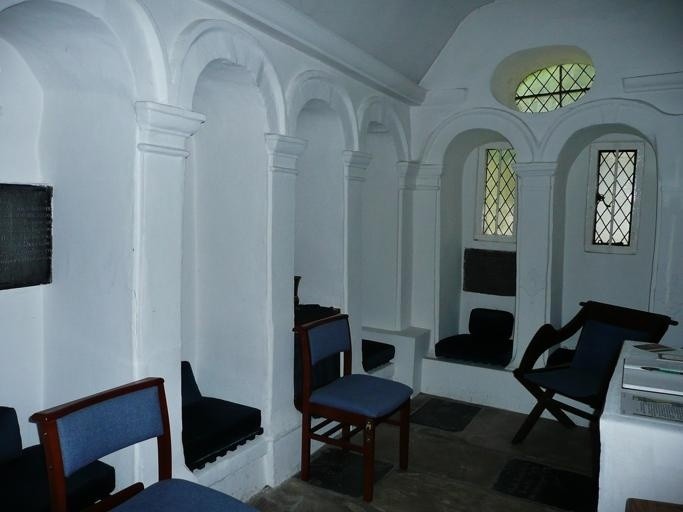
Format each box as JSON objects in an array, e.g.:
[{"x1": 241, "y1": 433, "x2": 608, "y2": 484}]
[{"x1": 641, "y1": 367, "x2": 683, "y2": 375}]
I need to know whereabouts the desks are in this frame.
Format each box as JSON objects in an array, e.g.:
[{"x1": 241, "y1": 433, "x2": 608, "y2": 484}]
[{"x1": 598, "y1": 340, "x2": 683, "y2": 512}]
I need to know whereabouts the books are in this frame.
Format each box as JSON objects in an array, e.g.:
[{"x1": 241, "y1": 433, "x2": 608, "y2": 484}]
[{"x1": 620, "y1": 356, "x2": 682, "y2": 397}]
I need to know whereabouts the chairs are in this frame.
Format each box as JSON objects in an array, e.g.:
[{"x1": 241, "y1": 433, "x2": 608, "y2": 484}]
[
  {"x1": 28, "y1": 378, "x2": 259, "y2": 512},
  {"x1": 0, "y1": 406, "x2": 115, "y2": 512},
  {"x1": 435, "y1": 307, "x2": 514, "y2": 410},
  {"x1": 181, "y1": 361, "x2": 267, "y2": 502},
  {"x1": 295, "y1": 314, "x2": 413, "y2": 502},
  {"x1": 363, "y1": 339, "x2": 395, "y2": 383},
  {"x1": 511, "y1": 300, "x2": 679, "y2": 446}
]
